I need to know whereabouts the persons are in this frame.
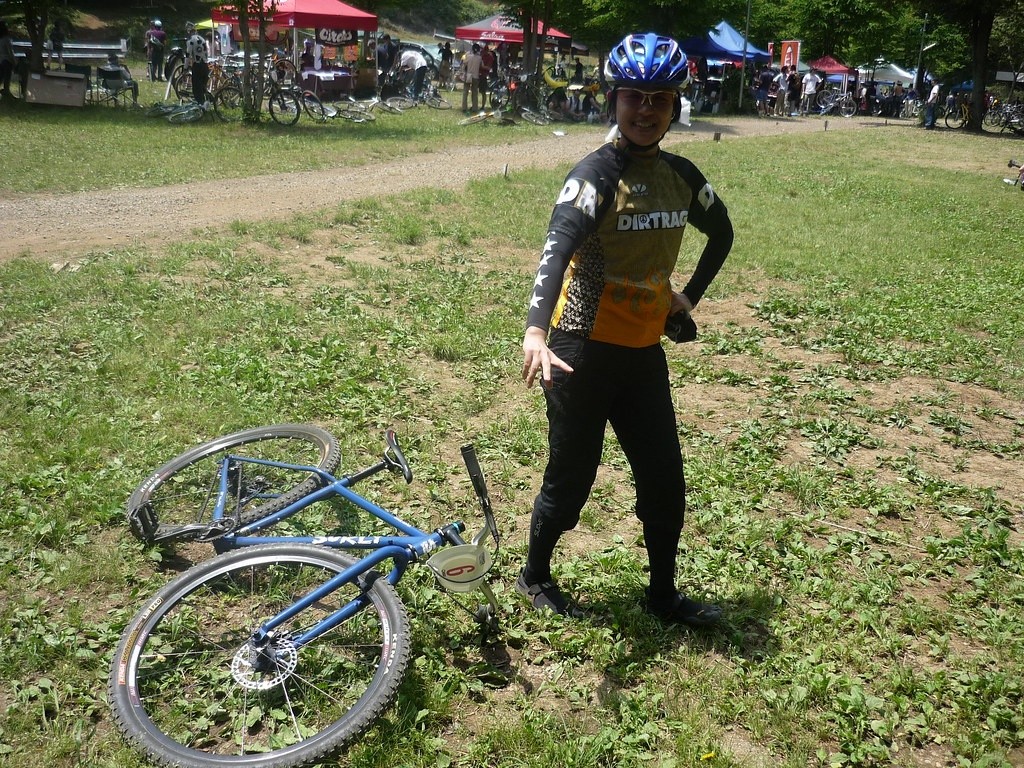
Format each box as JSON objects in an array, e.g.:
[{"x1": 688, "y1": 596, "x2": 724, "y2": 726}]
[
  {"x1": 46, "y1": 20, "x2": 64, "y2": 71},
  {"x1": 523, "y1": 33, "x2": 734, "y2": 635},
  {"x1": 1019, "y1": 164, "x2": 1024, "y2": 191},
  {"x1": 105, "y1": 54, "x2": 146, "y2": 109},
  {"x1": 183, "y1": 1, "x2": 1024, "y2": 128},
  {"x1": 17, "y1": 56, "x2": 26, "y2": 94},
  {"x1": 144, "y1": 20, "x2": 167, "y2": 82},
  {"x1": 0, "y1": 20, "x2": 15, "y2": 96}
]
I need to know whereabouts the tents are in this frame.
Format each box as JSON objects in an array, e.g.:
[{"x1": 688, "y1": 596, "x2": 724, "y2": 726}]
[
  {"x1": 950, "y1": 79, "x2": 987, "y2": 91},
  {"x1": 679, "y1": 21, "x2": 772, "y2": 110},
  {"x1": 205, "y1": 1, "x2": 379, "y2": 93},
  {"x1": 828, "y1": 55, "x2": 933, "y2": 90},
  {"x1": 455, "y1": 9, "x2": 574, "y2": 87},
  {"x1": 765, "y1": 57, "x2": 812, "y2": 77}
]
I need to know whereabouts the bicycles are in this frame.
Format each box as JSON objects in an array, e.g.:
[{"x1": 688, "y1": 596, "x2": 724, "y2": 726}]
[
  {"x1": 945, "y1": 100, "x2": 969, "y2": 129},
  {"x1": 146, "y1": 38, "x2": 453, "y2": 126},
  {"x1": 458, "y1": 66, "x2": 557, "y2": 126},
  {"x1": 107, "y1": 423, "x2": 500, "y2": 768},
  {"x1": 898, "y1": 98, "x2": 946, "y2": 120},
  {"x1": 983, "y1": 102, "x2": 1024, "y2": 135},
  {"x1": 816, "y1": 87, "x2": 856, "y2": 118}
]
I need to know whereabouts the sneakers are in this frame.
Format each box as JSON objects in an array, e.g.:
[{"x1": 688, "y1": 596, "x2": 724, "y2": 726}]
[
  {"x1": 644, "y1": 586, "x2": 722, "y2": 625},
  {"x1": 514, "y1": 566, "x2": 583, "y2": 621}
]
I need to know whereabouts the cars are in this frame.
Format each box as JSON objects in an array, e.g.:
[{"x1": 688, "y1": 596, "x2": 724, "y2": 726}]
[{"x1": 400, "y1": 41, "x2": 461, "y2": 81}]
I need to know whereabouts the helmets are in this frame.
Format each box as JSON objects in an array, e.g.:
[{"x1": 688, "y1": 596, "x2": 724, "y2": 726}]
[
  {"x1": 592, "y1": 84, "x2": 600, "y2": 90},
  {"x1": 150, "y1": 21, "x2": 155, "y2": 26},
  {"x1": 155, "y1": 21, "x2": 162, "y2": 27},
  {"x1": 604, "y1": 33, "x2": 690, "y2": 95}
]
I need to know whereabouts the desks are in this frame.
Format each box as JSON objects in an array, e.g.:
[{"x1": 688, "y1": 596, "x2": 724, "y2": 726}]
[{"x1": 304, "y1": 73, "x2": 352, "y2": 100}]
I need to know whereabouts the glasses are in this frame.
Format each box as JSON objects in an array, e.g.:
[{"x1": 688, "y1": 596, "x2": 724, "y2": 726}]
[{"x1": 616, "y1": 87, "x2": 676, "y2": 109}]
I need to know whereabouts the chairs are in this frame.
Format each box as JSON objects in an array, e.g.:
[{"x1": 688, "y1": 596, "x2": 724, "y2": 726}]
[
  {"x1": 97, "y1": 67, "x2": 136, "y2": 109},
  {"x1": 64, "y1": 63, "x2": 93, "y2": 103}
]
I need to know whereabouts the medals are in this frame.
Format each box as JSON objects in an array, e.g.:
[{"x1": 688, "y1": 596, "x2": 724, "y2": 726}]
[{"x1": 808, "y1": 55, "x2": 859, "y2": 115}]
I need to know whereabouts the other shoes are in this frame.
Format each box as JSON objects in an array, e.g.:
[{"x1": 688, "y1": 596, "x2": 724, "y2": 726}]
[
  {"x1": 46, "y1": 66, "x2": 50, "y2": 71},
  {"x1": 55, "y1": 67, "x2": 61, "y2": 70}
]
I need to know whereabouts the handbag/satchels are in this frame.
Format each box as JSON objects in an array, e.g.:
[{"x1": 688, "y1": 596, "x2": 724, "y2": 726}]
[{"x1": 47, "y1": 39, "x2": 53, "y2": 50}]
